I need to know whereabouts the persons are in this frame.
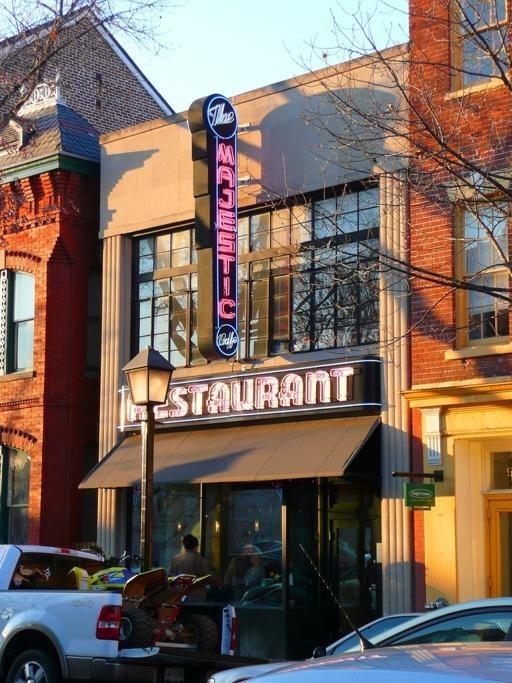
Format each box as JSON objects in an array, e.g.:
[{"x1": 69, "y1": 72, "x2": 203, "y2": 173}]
[
  {"x1": 244, "y1": 552, "x2": 267, "y2": 585},
  {"x1": 223, "y1": 544, "x2": 254, "y2": 588},
  {"x1": 168, "y1": 533, "x2": 207, "y2": 580}
]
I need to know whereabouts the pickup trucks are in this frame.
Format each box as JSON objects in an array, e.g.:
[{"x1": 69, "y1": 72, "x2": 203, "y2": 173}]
[{"x1": 1, "y1": 545, "x2": 238, "y2": 683}]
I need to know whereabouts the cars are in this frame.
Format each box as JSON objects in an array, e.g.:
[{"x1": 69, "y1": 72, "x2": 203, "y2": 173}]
[
  {"x1": 208, "y1": 641, "x2": 512, "y2": 683},
  {"x1": 344, "y1": 597, "x2": 512, "y2": 651},
  {"x1": 311, "y1": 611, "x2": 423, "y2": 655}
]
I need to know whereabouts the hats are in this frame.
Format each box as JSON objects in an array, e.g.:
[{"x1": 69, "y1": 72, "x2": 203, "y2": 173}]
[{"x1": 182, "y1": 534, "x2": 198, "y2": 550}]
